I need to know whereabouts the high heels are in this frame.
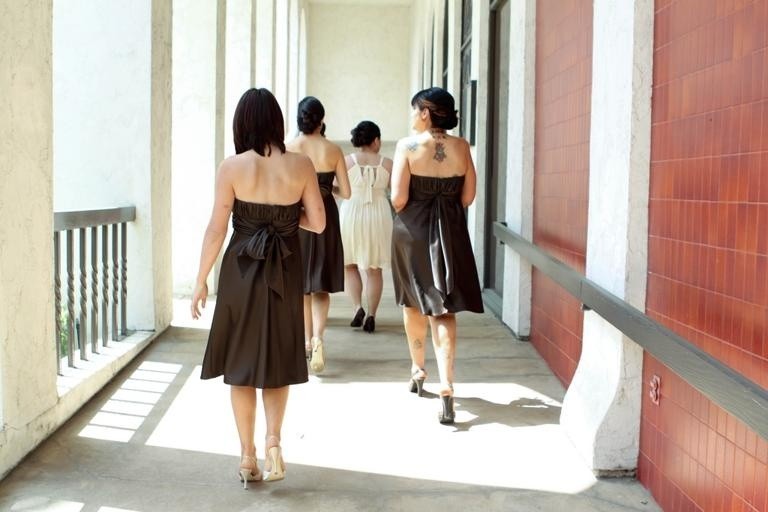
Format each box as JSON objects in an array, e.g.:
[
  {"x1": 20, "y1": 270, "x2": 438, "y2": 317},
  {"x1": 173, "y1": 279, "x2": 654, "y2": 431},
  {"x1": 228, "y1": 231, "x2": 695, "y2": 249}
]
[
  {"x1": 311, "y1": 336, "x2": 324, "y2": 372},
  {"x1": 238, "y1": 456, "x2": 261, "y2": 489},
  {"x1": 409, "y1": 369, "x2": 426, "y2": 397},
  {"x1": 351, "y1": 307, "x2": 365, "y2": 327},
  {"x1": 306, "y1": 342, "x2": 311, "y2": 361},
  {"x1": 263, "y1": 447, "x2": 285, "y2": 481},
  {"x1": 364, "y1": 316, "x2": 375, "y2": 333},
  {"x1": 438, "y1": 384, "x2": 454, "y2": 423}
]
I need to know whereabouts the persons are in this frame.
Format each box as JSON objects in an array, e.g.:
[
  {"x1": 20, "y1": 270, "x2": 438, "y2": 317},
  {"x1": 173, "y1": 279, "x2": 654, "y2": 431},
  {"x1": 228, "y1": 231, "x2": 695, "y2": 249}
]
[
  {"x1": 328, "y1": 120, "x2": 393, "y2": 334},
  {"x1": 283, "y1": 94, "x2": 353, "y2": 375},
  {"x1": 190, "y1": 86, "x2": 329, "y2": 493},
  {"x1": 386, "y1": 85, "x2": 486, "y2": 425}
]
